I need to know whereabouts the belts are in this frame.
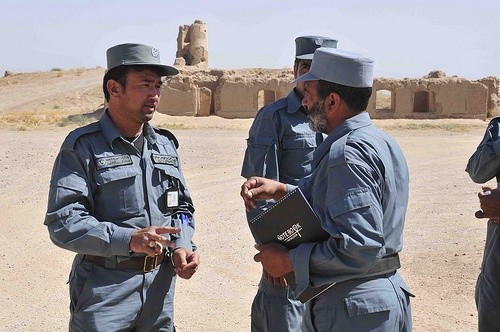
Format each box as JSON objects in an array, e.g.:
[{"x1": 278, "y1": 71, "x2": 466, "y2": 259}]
[{"x1": 78, "y1": 252, "x2": 166, "y2": 272}]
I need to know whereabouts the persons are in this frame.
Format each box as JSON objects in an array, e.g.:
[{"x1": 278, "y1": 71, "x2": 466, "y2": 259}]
[
  {"x1": 465, "y1": 116, "x2": 500, "y2": 332},
  {"x1": 44, "y1": 43, "x2": 200, "y2": 332},
  {"x1": 241, "y1": 46, "x2": 414, "y2": 332},
  {"x1": 242, "y1": 36, "x2": 340, "y2": 332}
]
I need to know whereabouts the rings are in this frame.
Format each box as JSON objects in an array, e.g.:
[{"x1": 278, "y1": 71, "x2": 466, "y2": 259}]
[{"x1": 149, "y1": 240, "x2": 157, "y2": 250}]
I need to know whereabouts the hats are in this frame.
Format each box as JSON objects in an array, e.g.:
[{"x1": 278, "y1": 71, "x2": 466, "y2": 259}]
[
  {"x1": 295, "y1": 35, "x2": 338, "y2": 60},
  {"x1": 289, "y1": 47, "x2": 375, "y2": 88},
  {"x1": 106, "y1": 42, "x2": 180, "y2": 77}
]
